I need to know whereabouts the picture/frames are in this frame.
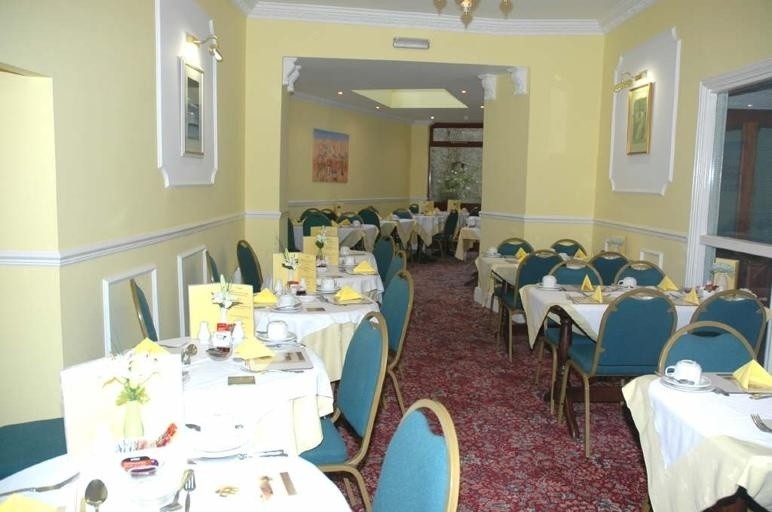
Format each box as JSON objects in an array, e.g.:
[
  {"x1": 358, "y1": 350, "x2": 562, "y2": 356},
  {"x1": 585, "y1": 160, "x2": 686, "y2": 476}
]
[
  {"x1": 625, "y1": 82, "x2": 652, "y2": 154},
  {"x1": 179, "y1": 56, "x2": 205, "y2": 158}
]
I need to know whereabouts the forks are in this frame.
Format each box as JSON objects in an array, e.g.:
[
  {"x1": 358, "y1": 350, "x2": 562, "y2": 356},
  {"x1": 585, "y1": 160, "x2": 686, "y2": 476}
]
[
  {"x1": 1, "y1": 470, "x2": 83, "y2": 499},
  {"x1": 183, "y1": 473, "x2": 197, "y2": 511},
  {"x1": 751, "y1": 414, "x2": 772, "y2": 434}
]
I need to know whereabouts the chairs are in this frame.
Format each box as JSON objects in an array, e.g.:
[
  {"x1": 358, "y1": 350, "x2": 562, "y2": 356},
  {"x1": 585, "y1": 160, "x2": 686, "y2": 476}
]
[
  {"x1": 0, "y1": 417, "x2": 66, "y2": 482},
  {"x1": 475, "y1": 236, "x2": 770, "y2": 457},
  {"x1": 285, "y1": 196, "x2": 480, "y2": 286},
  {"x1": 370, "y1": 398, "x2": 462, "y2": 509},
  {"x1": 124, "y1": 235, "x2": 415, "y2": 423},
  {"x1": 299, "y1": 309, "x2": 391, "y2": 511},
  {"x1": 658, "y1": 320, "x2": 771, "y2": 376}
]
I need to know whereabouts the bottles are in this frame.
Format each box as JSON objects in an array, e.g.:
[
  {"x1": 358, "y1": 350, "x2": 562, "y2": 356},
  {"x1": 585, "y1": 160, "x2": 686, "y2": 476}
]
[
  {"x1": 232, "y1": 266, "x2": 307, "y2": 294},
  {"x1": 203, "y1": 321, "x2": 232, "y2": 361},
  {"x1": 198, "y1": 320, "x2": 211, "y2": 343},
  {"x1": 234, "y1": 321, "x2": 244, "y2": 342}
]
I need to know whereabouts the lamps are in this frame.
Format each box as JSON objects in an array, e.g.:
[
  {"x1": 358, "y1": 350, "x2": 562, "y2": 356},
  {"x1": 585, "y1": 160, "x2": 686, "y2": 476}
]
[
  {"x1": 459, "y1": 0, "x2": 472, "y2": 15},
  {"x1": 612, "y1": 70, "x2": 648, "y2": 92},
  {"x1": 183, "y1": 33, "x2": 223, "y2": 63}
]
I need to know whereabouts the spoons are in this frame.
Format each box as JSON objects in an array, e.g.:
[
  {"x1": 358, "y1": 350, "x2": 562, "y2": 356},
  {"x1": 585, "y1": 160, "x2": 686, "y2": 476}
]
[
  {"x1": 746, "y1": 393, "x2": 771, "y2": 400},
  {"x1": 654, "y1": 370, "x2": 694, "y2": 385},
  {"x1": 239, "y1": 447, "x2": 287, "y2": 460},
  {"x1": 232, "y1": 353, "x2": 272, "y2": 363},
  {"x1": 185, "y1": 342, "x2": 198, "y2": 365},
  {"x1": 709, "y1": 388, "x2": 729, "y2": 398},
  {"x1": 160, "y1": 469, "x2": 194, "y2": 512},
  {"x1": 84, "y1": 478, "x2": 107, "y2": 512}
]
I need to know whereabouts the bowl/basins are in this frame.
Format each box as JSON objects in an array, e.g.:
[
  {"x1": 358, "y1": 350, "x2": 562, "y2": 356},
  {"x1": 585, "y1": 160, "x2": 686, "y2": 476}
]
[
  {"x1": 321, "y1": 279, "x2": 334, "y2": 288},
  {"x1": 297, "y1": 295, "x2": 317, "y2": 302}
]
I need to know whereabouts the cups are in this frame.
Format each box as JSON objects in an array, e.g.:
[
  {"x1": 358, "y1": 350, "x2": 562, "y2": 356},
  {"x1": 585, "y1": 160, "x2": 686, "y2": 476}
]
[
  {"x1": 664, "y1": 359, "x2": 703, "y2": 384},
  {"x1": 278, "y1": 294, "x2": 295, "y2": 306},
  {"x1": 267, "y1": 319, "x2": 289, "y2": 339},
  {"x1": 128, "y1": 463, "x2": 158, "y2": 483},
  {"x1": 341, "y1": 218, "x2": 362, "y2": 268},
  {"x1": 486, "y1": 245, "x2": 571, "y2": 263},
  {"x1": 617, "y1": 277, "x2": 637, "y2": 287},
  {"x1": 542, "y1": 275, "x2": 557, "y2": 288}
]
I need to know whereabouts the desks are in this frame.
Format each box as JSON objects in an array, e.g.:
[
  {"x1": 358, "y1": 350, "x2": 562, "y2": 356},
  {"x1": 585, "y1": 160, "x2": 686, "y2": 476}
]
[
  {"x1": 63, "y1": 333, "x2": 337, "y2": 465},
  {"x1": 621, "y1": 373, "x2": 771, "y2": 512}
]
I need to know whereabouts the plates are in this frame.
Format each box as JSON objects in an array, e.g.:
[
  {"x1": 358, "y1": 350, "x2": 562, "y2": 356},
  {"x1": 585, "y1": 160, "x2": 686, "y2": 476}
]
[
  {"x1": 257, "y1": 331, "x2": 296, "y2": 342},
  {"x1": 317, "y1": 287, "x2": 341, "y2": 293},
  {"x1": 271, "y1": 306, "x2": 302, "y2": 313},
  {"x1": 663, "y1": 372, "x2": 711, "y2": 391}
]
